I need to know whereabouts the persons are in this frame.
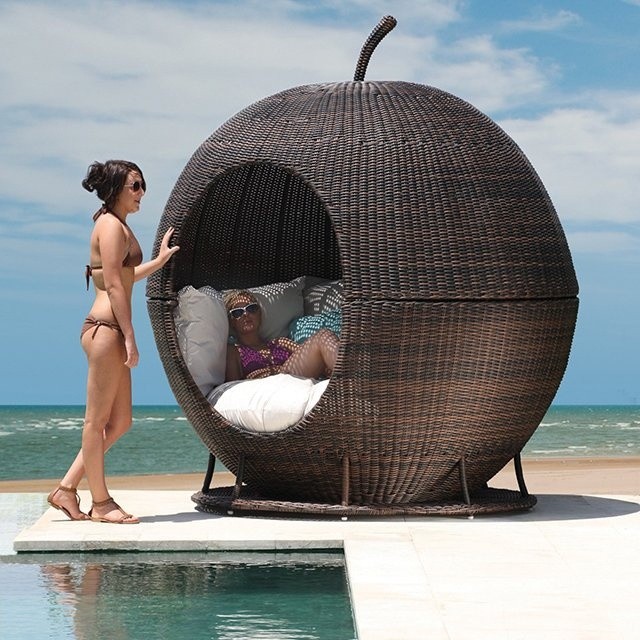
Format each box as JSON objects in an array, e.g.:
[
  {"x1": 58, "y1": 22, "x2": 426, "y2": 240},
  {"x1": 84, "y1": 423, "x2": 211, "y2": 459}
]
[
  {"x1": 47, "y1": 160, "x2": 179, "y2": 525},
  {"x1": 223, "y1": 290, "x2": 339, "y2": 381}
]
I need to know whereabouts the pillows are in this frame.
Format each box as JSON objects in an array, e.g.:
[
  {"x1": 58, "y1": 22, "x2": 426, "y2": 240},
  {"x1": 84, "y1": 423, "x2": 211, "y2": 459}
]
[
  {"x1": 289, "y1": 310, "x2": 341, "y2": 343},
  {"x1": 303, "y1": 277, "x2": 344, "y2": 312},
  {"x1": 173, "y1": 276, "x2": 304, "y2": 399}
]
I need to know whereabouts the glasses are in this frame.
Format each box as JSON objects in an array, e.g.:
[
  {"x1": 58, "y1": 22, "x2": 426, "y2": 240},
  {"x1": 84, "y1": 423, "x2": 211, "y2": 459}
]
[
  {"x1": 227, "y1": 302, "x2": 260, "y2": 320},
  {"x1": 123, "y1": 181, "x2": 147, "y2": 192}
]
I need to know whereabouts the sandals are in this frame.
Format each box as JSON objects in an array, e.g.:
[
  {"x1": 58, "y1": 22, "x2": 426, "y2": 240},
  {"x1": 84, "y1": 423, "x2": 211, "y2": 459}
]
[
  {"x1": 46, "y1": 483, "x2": 92, "y2": 521},
  {"x1": 87, "y1": 497, "x2": 140, "y2": 525}
]
